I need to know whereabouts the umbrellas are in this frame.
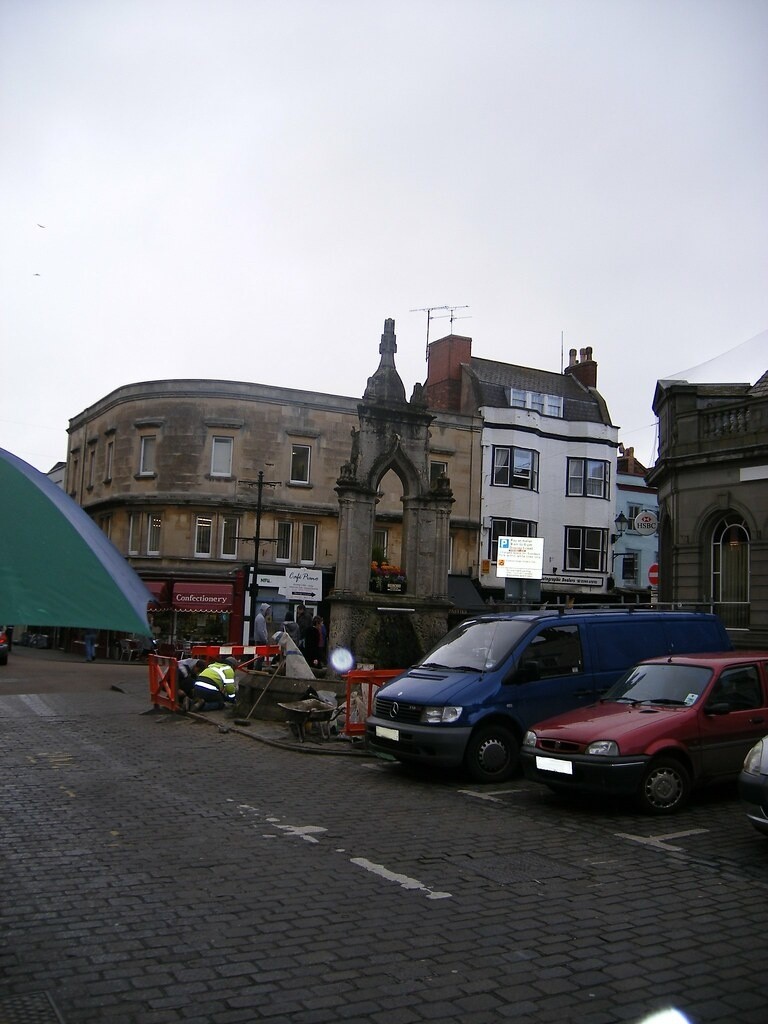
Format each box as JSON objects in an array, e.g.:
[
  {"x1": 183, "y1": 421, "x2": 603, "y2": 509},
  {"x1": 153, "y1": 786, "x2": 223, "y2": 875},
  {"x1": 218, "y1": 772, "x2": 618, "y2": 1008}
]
[{"x1": 0, "y1": 447, "x2": 158, "y2": 638}]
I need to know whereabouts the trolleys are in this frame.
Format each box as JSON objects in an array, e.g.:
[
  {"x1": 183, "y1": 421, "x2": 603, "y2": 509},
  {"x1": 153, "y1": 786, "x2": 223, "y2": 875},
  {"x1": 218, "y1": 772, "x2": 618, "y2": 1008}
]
[{"x1": 277, "y1": 699, "x2": 346, "y2": 744}]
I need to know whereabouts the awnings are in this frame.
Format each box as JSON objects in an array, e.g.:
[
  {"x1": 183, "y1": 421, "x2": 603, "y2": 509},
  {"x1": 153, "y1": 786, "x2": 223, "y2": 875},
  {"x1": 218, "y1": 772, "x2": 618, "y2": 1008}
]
[
  {"x1": 172, "y1": 583, "x2": 233, "y2": 613},
  {"x1": 142, "y1": 582, "x2": 171, "y2": 612}
]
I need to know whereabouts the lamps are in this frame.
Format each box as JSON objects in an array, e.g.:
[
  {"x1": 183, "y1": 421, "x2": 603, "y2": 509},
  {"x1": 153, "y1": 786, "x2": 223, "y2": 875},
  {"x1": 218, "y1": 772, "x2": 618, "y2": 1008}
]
[{"x1": 611, "y1": 510, "x2": 630, "y2": 544}]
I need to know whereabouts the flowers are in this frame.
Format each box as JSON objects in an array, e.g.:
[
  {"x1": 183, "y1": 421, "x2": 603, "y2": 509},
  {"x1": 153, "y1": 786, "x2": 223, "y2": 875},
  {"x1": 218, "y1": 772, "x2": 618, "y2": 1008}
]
[{"x1": 371, "y1": 561, "x2": 406, "y2": 575}]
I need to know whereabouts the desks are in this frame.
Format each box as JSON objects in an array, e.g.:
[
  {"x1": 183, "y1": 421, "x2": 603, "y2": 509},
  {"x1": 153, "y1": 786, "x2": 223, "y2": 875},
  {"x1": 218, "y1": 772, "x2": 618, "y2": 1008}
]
[{"x1": 178, "y1": 641, "x2": 223, "y2": 659}]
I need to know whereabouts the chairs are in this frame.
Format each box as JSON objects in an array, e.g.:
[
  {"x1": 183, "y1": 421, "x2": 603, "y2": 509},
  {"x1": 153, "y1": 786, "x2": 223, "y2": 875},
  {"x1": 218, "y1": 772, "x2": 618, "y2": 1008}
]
[
  {"x1": 181, "y1": 640, "x2": 193, "y2": 660},
  {"x1": 119, "y1": 639, "x2": 139, "y2": 663}
]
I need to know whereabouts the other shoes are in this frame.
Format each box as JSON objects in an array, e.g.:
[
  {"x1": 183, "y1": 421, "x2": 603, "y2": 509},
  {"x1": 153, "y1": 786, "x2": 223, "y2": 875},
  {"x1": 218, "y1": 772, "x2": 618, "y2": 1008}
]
[
  {"x1": 183, "y1": 697, "x2": 189, "y2": 712},
  {"x1": 191, "y1": 699, "x2": 205, "y2": 711}
]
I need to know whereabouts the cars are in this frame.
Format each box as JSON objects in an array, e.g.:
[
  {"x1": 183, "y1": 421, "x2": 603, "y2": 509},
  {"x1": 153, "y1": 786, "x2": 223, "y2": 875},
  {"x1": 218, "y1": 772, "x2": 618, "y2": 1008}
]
[{"x1": 521, "y1": 650, "x2": 768, "y2": 837}]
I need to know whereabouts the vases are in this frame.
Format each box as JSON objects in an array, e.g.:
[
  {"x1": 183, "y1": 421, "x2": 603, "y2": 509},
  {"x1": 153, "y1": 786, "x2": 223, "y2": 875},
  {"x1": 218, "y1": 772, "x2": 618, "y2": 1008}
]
[{"x1": 371, "y1": 575, "x2": 407, "y2": 593}]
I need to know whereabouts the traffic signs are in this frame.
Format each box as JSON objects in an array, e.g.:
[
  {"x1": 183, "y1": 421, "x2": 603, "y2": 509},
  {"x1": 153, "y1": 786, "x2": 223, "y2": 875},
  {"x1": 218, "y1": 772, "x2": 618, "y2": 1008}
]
[{"x1": 285, "y1": 569, "x2": 322, "y2": 601}]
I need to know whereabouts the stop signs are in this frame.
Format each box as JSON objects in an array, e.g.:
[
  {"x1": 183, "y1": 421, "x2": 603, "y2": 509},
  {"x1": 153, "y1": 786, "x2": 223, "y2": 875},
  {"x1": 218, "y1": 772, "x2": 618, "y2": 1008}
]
[{"x1": 648, "y1": 565, "x2": 658, "y2": 585}]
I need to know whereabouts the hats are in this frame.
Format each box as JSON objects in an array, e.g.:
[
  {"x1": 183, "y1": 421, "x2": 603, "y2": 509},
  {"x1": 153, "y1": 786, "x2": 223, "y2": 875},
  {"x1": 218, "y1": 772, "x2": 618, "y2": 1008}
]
[{"x1": 226, "y1": 657, "x2": 238, "y2": 666}]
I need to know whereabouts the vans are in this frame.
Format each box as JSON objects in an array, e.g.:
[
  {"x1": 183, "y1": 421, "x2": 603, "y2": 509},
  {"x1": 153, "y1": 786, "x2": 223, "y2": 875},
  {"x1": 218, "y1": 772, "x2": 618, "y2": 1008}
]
[{"x1": 364, "y1": 603, "x2": 734, "y2": 786}]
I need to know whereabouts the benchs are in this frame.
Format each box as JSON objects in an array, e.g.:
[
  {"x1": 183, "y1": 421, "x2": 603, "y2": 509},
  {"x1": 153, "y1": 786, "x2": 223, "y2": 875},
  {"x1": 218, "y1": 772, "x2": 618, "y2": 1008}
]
[{"x1": 175, "y1": 649, "x2": 192, "y2": 661}]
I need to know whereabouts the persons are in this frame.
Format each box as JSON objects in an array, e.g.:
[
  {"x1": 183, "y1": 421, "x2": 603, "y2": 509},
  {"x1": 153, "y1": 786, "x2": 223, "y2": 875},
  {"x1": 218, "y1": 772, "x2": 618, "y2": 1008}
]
[
  {"x1": 5, "y1": 626, "x2": 13, "y2": 651},
  {"x1": 84, "y1": 629, "x2": 96, "y2": 661},
  {"x1": 162, "y1": 656, "x2": 238, "y2": 712},
  {"x1": 254, "y1": 603, "x2": 271, "y2": 670},
  {"x1": 140, "y1": 624, "x2": 153, "y2": 661},
  {"x1": 280, "y1": 612, "x2": 300, "y2": 647},
  {"x1": 297, "y1": 605, "x2": 328, "y2": 668}
]
